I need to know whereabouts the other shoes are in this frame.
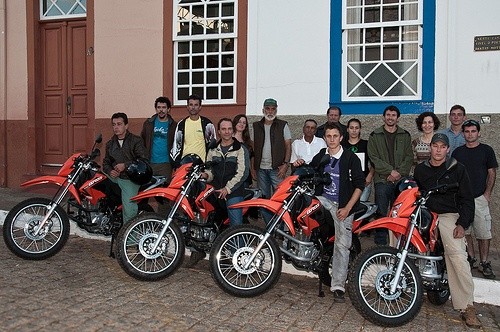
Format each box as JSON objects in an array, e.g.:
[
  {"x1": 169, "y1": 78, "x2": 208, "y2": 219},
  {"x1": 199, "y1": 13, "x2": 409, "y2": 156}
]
[
  {"x1": 462, "y1": 305, "x2": 483, "y2": 328},
  {"x1": 333, "y1": 289, "x2": 346, "y2": 303},
  {"x1": 468, "y1": 256, "x2": 479, "y2": 268},
  {"x1": 478, "y1": 260, "x2": 496, "y2": 279},
  {"x1": 186, "y1": 251, "x2": 207, "y2": 269}
]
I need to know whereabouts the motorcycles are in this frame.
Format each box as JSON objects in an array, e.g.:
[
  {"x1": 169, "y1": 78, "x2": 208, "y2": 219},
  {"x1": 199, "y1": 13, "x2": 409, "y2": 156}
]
[
  {"x1": 346, "y1": 177, "x2": 479, "y2": 328},
  {"x1": 205, "y1": 153, "x2": 381, "y2": 297},
  {"x1": 107, "y1": 138, "x2": 263, "y2": 282},
  {"x1": 2, "y1": 133, "x2": 168, "y2": 260}
]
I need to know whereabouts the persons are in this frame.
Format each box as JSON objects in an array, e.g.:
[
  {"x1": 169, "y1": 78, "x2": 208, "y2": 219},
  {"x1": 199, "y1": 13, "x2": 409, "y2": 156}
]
[
  {"x1": 292, "y1": 123, "x2": 365, "y2": 302},
  {"x1": 339, "y1": 118, "x2": 374, "y2": 237},
  {"x1": 366, "y1": 106, "x2": 413, "y2": 247},
  {"x1": 290, "y1": 119, "x2": 327, "y2": 176},
  {"x1": 413, "y1": 134, "x2": 482, "y2": 327},
  {"x1": 230, "y1": 114, "x2": 253, "y2": 164},
  {"x1": 250, "y1": 98, "x2": 292, "y2": 225},
  {"x1": 450, "y1": 120, "x2": 498, "y2": 279},
  {"x1": 169, "y1": 94, "x2": 216, "y2": 173},
  {"x1": 141, "y1": 96, "x2": 178, "y2": 185},
  {"x1": 410, "y1": 111, "x2": 440, "y2": 176},
  {"x1": 187, "y1": 118, "x2": 249, "y2": 269},
  {"x1": 102, "y1": 112, "x2": 149, "y2": 252},
  {"x1": 315, "y1": 106, "x2": 348, "y2": 141},
  {"x1": 443, "y1": 105, "x2": 466, "y2": 159}
]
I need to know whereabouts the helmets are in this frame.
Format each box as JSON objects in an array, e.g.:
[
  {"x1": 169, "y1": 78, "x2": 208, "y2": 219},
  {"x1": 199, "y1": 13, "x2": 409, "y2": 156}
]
[
  {"x1": 127, "y1": 160, "x2": 153, "y2": 185},
  {"x1": 394, "y1": 177, "x2": 419, "y2": 199}
]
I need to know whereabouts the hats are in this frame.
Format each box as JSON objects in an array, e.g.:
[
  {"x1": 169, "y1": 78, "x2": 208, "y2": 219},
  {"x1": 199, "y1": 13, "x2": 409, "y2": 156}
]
[
  {"x1": 263, "y1": 98, "x2": 278, "y2": 107},
  {"x1": 430, "y1": 133, "x2": 450, "y2": 148}
]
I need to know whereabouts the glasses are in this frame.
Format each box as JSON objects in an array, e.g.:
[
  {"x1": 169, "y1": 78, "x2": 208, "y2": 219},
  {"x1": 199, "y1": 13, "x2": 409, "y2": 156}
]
[{"x1": 463, "y1": 120, "x2": 478, "y2": 125}]
